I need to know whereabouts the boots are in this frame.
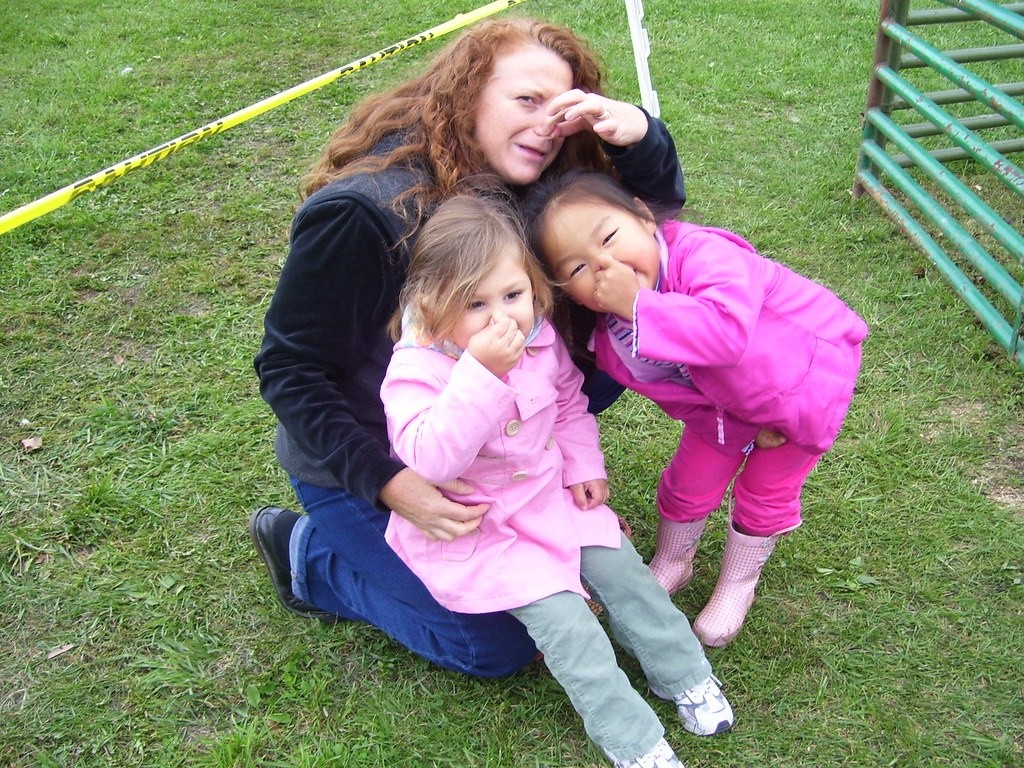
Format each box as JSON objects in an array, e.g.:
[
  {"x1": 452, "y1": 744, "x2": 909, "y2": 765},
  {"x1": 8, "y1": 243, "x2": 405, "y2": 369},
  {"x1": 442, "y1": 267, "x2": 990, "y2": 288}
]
[
  {"x1": 692, "y1": 490, "x2": 802, "y2": 647},
  {"x1": 648, "y1": 513, "x2": 709, "y2": 596}
]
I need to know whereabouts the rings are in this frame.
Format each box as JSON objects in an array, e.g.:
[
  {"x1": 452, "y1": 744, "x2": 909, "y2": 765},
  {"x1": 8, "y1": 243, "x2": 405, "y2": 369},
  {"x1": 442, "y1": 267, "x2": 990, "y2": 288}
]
[{"x1": 591, "y1": 109, "x2": 608, "y2": 120}]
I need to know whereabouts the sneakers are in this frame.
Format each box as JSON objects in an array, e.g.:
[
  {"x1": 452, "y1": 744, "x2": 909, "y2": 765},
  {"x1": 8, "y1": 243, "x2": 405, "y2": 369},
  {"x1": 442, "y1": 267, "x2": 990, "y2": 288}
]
[
  {"x1": 648, "y1": 672, "x2": 735, "y2": 736},
  {"x1": 604, "y1": 737, "x2": 685, "y2": 768}
]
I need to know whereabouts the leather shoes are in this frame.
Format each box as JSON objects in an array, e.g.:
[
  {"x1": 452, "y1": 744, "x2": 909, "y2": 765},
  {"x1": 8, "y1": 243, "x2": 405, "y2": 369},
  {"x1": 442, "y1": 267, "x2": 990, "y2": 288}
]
[{"x1": 249, "y1": 505, "x2": 341, "y2": 623}]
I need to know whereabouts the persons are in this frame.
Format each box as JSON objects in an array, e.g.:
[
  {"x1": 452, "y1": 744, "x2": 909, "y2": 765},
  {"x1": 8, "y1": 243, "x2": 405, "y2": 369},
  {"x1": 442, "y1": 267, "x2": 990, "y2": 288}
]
[
  {"x1": 379, "y1": 194, "x2": 733, "y2": 767},
  {"x1": 248, "y1": 16, "x2": 688, "y2": 683},
  {"x1": 520, "y1": 172, "x2": 867, "y2": 648}
]
